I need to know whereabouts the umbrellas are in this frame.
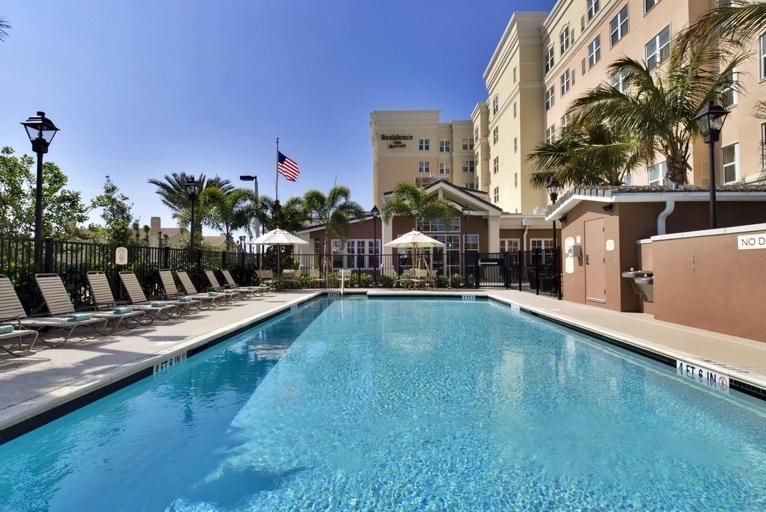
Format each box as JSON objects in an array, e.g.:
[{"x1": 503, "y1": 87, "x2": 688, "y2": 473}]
[
  {"x1": 247, "y1": 229, "x2": 310, "y2": 290},
  {"x1": 382, "y1": 228, "x2": 447, "y2": 277}
]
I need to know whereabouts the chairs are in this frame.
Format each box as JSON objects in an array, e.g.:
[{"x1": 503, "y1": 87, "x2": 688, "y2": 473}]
[
  {"x1": 257, "y1": 268, "x2": 353, "y2": 290},
  {"x1": 0, "y1": 269, "x2": 268, "y2": 358},
  {"x1": 395, "y1": 268, "x2": 438, "y2": 290}
]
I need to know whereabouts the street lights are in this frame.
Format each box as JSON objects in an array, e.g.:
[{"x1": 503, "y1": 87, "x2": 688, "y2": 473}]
[
  {"x1": 19, "y1": 111, "x2": 60, "y2": 273},
  {"x1": 545, "y1": 174, "x2": 564, "y2": 294},
  {"x1": 461, "y1": 203, "x2": 472, "y2": 289},
  {"x1": 370, "y1": 205, "x2": 380, "y2": 287},
  {"x1": 690, "y1": 100, "x2": 731, "y2": 230},
  {"x1": 239, "y1": 175, "x2": 260, "y2": 268},
  {"x1": 182, "y1": 177, "x2": 203, "y2": 269}
]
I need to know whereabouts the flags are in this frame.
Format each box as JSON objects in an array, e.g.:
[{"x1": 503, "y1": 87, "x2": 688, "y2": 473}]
[{"x1": 276, "y1": 149, "x2": 301, "y2": 184}]
